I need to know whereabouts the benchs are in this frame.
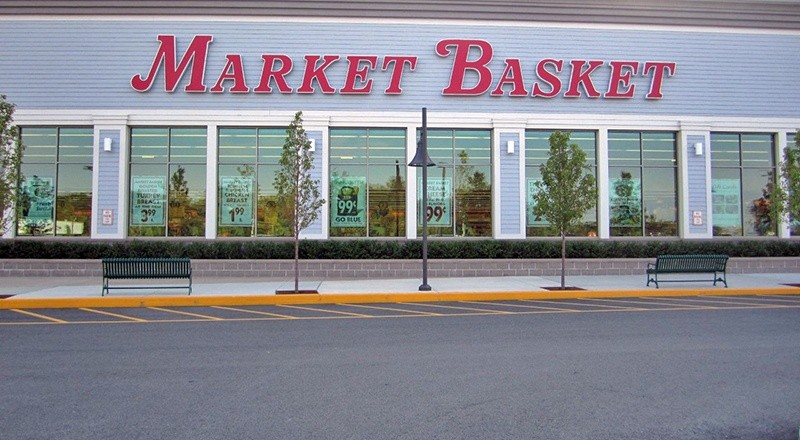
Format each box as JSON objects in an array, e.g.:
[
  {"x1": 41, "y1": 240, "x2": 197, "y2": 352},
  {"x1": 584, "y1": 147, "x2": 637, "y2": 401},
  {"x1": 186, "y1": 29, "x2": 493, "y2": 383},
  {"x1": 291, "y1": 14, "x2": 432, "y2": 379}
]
[
  {"x1": 646, "y1": 254, "x2": 730, "y2": 289},
  {"x1": 101, "y1": 257, "x2": 194, "y2": 296}
]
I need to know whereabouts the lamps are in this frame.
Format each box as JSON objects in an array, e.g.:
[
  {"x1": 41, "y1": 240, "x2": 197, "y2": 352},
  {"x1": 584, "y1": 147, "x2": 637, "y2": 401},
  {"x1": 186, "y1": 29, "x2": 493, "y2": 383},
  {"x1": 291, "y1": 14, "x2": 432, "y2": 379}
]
[
  {"x1": 507, "y1": 140, "x2": 515, "y2": 154},
  {"x1": 103, "y1": 137, "x2": 112, "y2": 152},
  {"x1": 307, "y1": 138, "x2": 315, "y2": 152},
  {"x1": 694, "y1": 143, "x2": 702, "y2": 155}
]
